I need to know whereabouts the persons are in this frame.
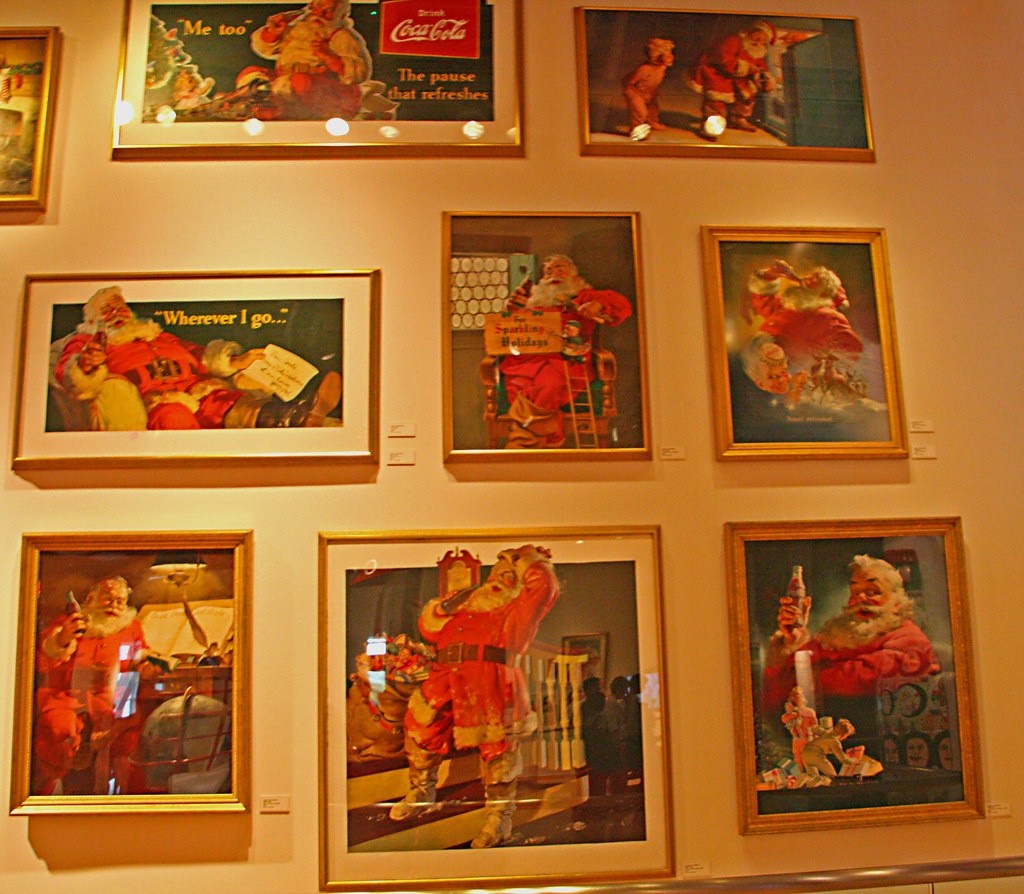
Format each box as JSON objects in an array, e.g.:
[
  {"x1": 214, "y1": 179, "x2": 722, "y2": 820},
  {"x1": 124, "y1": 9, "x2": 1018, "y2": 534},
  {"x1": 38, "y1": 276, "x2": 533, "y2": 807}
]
[{"x1": 566, "y1": 673, "x2": 643, "y2": 794}]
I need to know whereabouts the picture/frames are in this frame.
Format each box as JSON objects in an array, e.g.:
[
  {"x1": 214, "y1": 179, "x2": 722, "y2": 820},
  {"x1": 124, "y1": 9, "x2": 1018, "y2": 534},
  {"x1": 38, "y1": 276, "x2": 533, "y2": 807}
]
[
  {"x1": 14, "y1": 269, "x2": 382, "y2": 487},
  {"x1": 441, "y1": 210, "x2": 653, "y2": 464},
  {"x1": 0, "y1": 27, "x2": 64, "y2": 211},
  {"x1": 700, "y1": 222, "x2": 913, "y2": 462},
  {"x1": 722, "y1": 514, "x2": 986, "y2": 836},
  {"x1": 8, "y1": 528, "x2": 253, "y2": 817},
  {"x1": 111, "y1": 0, "x2": 526, "y2": 159},
  {"x1": 573, "y1": 5, "x2": 876, "y2": 164},
  {"x1": 316, "y1": 524, "x2": 677, "y2": 894}
]
